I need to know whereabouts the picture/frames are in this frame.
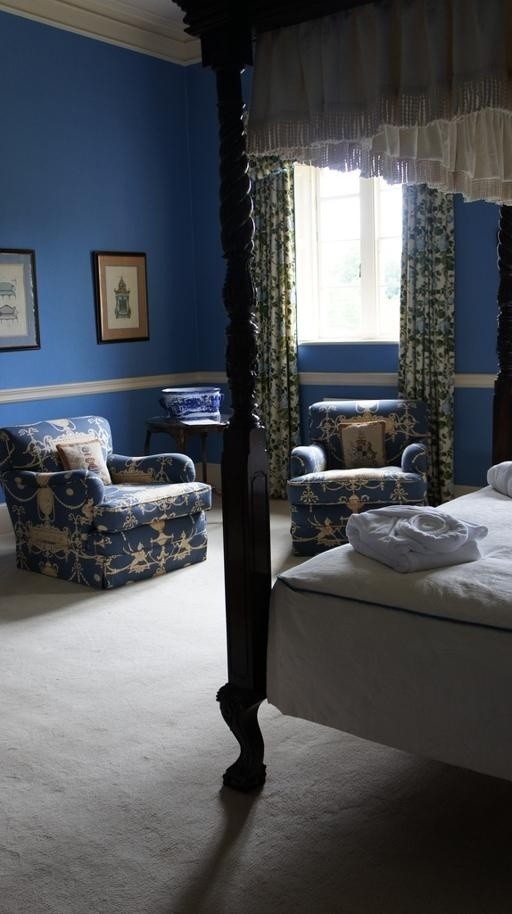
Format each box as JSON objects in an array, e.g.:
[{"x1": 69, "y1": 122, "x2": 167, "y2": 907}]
[
  {"x1": 0, "y1": 246, "x2": 42, "y2": 352},
  {"x1": 91, "y1": 249, "x2": 151, "y2": 345}
]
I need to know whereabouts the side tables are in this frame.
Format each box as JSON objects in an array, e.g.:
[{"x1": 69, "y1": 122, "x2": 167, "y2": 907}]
[{"x1": 143, "y1": 413, "x2": 230, "y2": 495}]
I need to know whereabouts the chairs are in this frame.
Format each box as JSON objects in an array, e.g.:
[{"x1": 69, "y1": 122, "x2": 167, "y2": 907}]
[
  {"x1": 1, "y1": 413, "x2": 214, "y2": 592},
  {"x1": 286, "y1": 397, "x2": 428, "y2": 557}
]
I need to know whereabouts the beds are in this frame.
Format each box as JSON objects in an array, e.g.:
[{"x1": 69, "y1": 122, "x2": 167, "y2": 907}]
[{"x1": 216, "y1": 482, "x2": 511, "y2": 796}]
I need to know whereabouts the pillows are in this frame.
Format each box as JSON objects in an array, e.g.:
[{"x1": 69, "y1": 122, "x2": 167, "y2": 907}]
[
  {"x1": 56, "y1": 438, "x2": 113, "y2": 487},
  {"x1": 337, "y1": 420, "x2": 387, "y2": 469}
]
